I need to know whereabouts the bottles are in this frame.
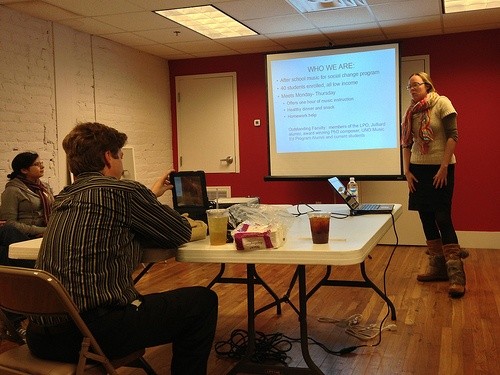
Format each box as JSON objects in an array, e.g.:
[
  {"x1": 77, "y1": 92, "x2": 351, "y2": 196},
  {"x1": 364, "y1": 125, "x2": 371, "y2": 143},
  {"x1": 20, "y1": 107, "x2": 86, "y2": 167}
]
[{"x1": 347, "y1": 177, "x2": 359, "y2": 203}]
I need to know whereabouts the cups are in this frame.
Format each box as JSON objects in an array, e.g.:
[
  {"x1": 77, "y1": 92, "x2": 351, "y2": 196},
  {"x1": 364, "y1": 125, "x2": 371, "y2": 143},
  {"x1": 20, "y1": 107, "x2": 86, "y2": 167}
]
[
  {"x1": 307, "y1": 211, "x2": 331, "y2": 244},
  {"x1": 206, "y1": 208, "x2": 228, "y2": 245}
]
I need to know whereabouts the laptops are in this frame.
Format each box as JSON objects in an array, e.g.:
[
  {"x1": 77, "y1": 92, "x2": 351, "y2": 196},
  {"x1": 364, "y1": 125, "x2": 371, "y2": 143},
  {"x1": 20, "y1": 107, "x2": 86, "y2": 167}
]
[
  {"x1": 171, "y1": 171, "x2": 210, "y2": 224},
  {"x1": 327, "y1": 176, "x2": 394, "y2": 216}
]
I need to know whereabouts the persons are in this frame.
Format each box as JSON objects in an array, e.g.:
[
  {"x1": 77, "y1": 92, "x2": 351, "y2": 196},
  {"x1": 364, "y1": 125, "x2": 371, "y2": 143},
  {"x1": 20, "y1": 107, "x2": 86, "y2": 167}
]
[
  {"x1": 25, "y1": 122, "x2": 219, "y2": 375},
  {"x1": 401, "y1": 72, "x2": 470, "y2": 297},
  {"x1": 0, "y1": 220, "x2": 37, "y2": 342},
  {"x1": 2, "y1": 151, "x2": 58, "y2": 241}
]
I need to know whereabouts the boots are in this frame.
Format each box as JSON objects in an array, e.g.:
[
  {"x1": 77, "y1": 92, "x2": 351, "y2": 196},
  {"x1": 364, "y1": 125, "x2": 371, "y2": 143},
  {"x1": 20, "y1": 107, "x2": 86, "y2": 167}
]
[
  {"x1": 417, "y1": 239, "x2": 450, "y2": 281},
  {"x1": 442, "y1": 244, "x2": 470, "y2": 296}
]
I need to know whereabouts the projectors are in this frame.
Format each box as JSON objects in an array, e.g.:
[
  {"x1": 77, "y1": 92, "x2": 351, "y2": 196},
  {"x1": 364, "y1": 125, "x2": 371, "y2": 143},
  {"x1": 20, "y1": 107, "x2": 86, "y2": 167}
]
[{"x1": 214, "y1": 197, "x2": 259, "y2": 211}]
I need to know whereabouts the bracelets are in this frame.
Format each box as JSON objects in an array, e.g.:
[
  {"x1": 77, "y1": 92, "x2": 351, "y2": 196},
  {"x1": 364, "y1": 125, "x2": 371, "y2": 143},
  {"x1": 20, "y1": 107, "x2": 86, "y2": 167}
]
[{"x1": 439, "y1": 166, "x2": 447, "y2": 168}]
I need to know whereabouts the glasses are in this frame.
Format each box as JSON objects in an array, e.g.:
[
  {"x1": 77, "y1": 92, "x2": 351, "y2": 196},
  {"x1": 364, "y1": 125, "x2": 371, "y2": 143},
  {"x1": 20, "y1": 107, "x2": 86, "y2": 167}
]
[
  {"x1": 31, "y1": 161, "x2": 43, "y2": 167},
  {"x1": 407, "y1": 82, "x2": 427, "y2": 90}
]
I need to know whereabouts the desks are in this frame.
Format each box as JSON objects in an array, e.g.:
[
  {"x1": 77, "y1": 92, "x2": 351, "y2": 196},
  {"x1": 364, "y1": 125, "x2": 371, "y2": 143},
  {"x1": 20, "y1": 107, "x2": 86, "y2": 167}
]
[{"x1": 9, "y1": 203, "x2": 403, "y2": 375}]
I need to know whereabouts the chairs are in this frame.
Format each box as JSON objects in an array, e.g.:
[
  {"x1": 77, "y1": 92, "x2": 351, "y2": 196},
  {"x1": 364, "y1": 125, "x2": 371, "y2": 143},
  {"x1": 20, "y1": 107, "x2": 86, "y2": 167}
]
[{"x1": 0, "y1": 265, "x2": 155, "y2": 375}]
